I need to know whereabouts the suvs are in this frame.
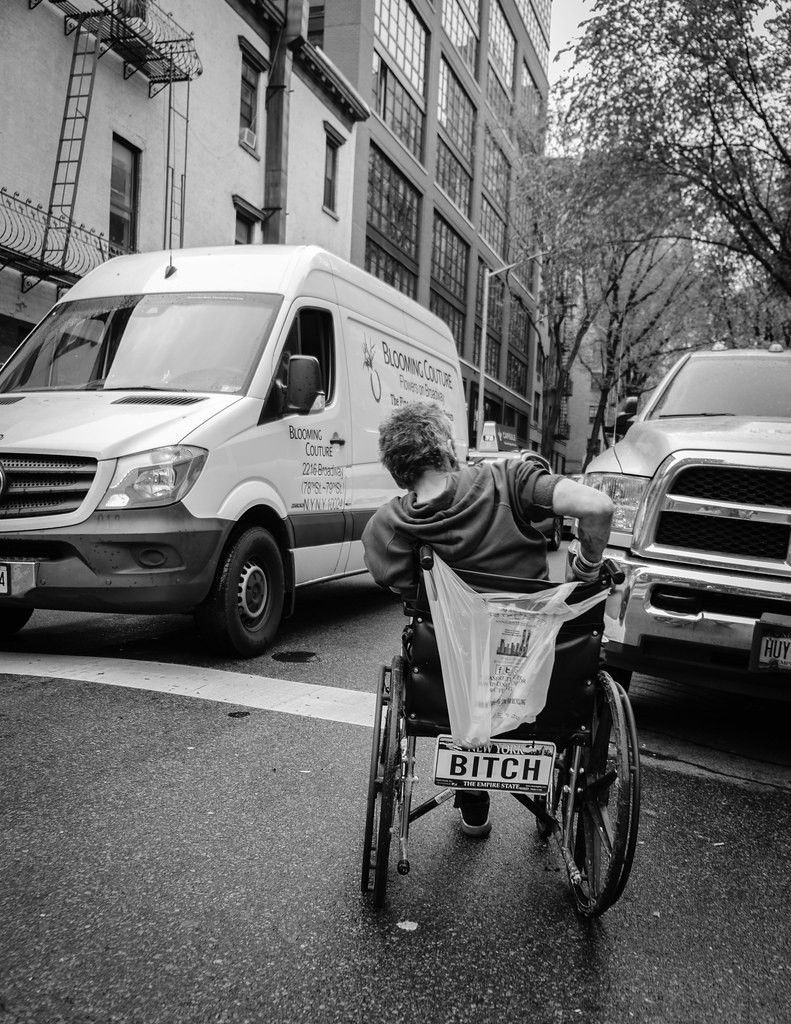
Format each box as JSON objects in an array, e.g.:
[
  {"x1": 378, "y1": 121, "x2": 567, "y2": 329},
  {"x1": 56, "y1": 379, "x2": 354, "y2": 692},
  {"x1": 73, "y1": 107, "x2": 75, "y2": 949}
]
[{"x1": 560, "y1": 341, "x2": 791, "y2": 704}]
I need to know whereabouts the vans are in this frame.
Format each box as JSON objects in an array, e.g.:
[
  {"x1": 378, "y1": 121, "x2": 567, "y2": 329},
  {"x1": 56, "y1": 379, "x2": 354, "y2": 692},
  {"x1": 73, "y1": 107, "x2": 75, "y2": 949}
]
[{"x1": 1, "y1": 243, "x2": 470, "y2": 668}]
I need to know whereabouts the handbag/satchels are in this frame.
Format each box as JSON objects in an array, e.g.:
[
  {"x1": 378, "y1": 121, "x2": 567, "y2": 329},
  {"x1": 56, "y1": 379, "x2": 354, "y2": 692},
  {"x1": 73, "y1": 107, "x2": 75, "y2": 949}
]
[{"x1": 422, "y1": 552, "x2": 611, "y2": 749}]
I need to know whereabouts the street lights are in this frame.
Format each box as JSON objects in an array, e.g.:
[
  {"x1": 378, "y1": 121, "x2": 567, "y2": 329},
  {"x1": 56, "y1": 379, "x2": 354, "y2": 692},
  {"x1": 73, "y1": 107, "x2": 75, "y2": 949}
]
[{"x1": 476, "y1": 245, "x2": 558, "y2": 454}]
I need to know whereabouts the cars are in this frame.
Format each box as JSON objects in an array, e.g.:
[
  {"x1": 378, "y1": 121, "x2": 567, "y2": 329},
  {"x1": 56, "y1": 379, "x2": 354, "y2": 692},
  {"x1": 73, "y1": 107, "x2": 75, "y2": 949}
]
[{"x1": 468, "y1": 420, "x2": 584, "y2": 554}]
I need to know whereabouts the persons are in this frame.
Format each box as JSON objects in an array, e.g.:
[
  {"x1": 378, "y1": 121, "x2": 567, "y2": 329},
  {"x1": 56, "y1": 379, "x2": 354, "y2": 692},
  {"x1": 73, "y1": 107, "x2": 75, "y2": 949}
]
[
  {"x1": 276, "y1": 341, "x2": 305, "y2": 384},
  {"x1": 364, "y1": 403, "x2": 615, "y2": 837}
]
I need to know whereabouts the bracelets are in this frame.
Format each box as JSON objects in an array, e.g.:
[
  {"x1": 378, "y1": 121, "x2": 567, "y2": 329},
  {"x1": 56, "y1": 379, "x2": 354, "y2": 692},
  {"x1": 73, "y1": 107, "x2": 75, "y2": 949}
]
[{"x1": 570, "y1": 548, "x2": 602, "y2": 581}]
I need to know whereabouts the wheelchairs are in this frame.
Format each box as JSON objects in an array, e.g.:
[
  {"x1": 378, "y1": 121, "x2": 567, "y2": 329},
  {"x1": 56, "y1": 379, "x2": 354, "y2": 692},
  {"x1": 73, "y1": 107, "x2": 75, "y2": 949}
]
[{"x1": 360, "y1": 558, "x2": 643, "y2": 921}]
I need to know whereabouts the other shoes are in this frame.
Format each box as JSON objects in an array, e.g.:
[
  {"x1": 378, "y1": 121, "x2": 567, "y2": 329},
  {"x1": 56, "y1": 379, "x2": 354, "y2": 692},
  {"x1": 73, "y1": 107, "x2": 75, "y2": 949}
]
[{"x1": 453, "y1": 790, "x2": 491, "y2": 834}]
